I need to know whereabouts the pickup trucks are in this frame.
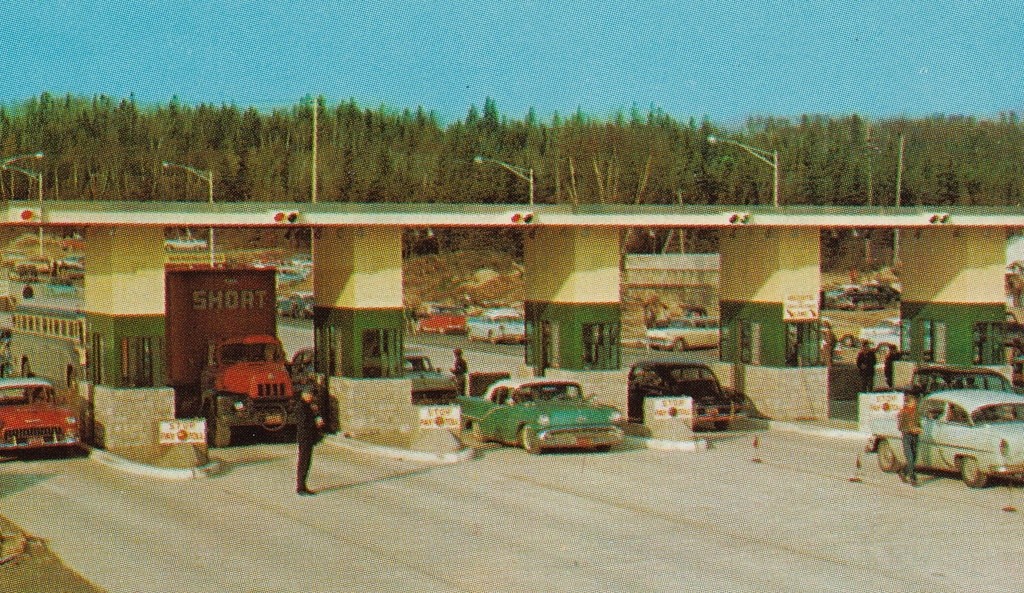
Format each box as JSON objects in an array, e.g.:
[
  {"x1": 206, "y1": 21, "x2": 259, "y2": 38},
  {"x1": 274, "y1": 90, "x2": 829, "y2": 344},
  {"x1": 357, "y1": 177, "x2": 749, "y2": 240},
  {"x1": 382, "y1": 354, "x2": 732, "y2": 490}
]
[{"x1": 872, "y1": 389, "x2": 1024, "y2": 489}]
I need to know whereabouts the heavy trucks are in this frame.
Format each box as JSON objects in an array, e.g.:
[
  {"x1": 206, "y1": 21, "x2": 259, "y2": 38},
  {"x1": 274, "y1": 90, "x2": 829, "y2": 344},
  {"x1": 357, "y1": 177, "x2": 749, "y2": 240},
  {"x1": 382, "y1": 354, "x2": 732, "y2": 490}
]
[{"x1": 12, "y1": 261, "x2": 299, "y2": 447}]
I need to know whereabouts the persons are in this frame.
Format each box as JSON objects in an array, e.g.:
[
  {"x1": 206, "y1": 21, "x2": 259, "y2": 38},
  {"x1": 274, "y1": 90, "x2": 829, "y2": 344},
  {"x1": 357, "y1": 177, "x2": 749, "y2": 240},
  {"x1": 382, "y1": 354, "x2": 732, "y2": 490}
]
[
  {"x1": 290, "y1": 298, "x2": 296, "y2": 319},
  {"x1": 642, "y1": 295, "x2": 709, "y2": 329},
  {"x1": 856, "y1": 341, "x2": 877, "y2": 392},
  {"x1": 885, "y1": 345, "x2": 902, "y2": 388},
  {"x1": 0, "y1": 342, "x2": 12, "y2": 378},
  {"x1": 295, "y1": 386, "x2": 324, "y2": 496},
  {"x1": 19, "y1": 257, "x2": 67, "y2": 300},
  {"x1": 822, "y1": 320, "x2": 836, "y2": 368},
  {"x1": 898, "y1": 395, "x2": 923, "y2": 487},
  {"x1": 450, "y1": 349, "x2": 468, "y2": 396},
  {"x1": 304, "y1": 300, "x2": 311, "y2": 319},
  {"x1": 403, "y1": 303, "x2": 418, "y2": 336}
]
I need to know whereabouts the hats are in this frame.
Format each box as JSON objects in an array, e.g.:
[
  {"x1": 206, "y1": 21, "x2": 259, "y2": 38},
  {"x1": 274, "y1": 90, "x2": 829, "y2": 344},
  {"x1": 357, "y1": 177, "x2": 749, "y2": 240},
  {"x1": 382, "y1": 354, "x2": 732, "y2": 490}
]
[
  {"x1": 302, "y1": 386, "x2": 314, "y2": 393},
  {"x1": 452, "y1": 348, "x2": 463, "y2": 355}
]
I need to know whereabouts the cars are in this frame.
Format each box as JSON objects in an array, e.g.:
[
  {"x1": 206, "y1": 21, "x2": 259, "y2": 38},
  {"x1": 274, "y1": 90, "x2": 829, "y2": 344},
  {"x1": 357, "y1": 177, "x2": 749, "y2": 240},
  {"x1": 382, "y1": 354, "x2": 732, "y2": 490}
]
[
  {"x1": 452, "y1": 378, "x2": 625, "y2": 456},
  {"x1": 878, "y1": 366, "x2": 1015, "y2": 394},
  {"x1": 285, "y1": 346, "x2": 319, "y2": 375},
  {"x1": 0, "y1": 376, "x2": 82, "y2": 456},
  {"x1": 416, "y1": 304, "x2": 468, "y2": 335},
  {"x1": 275, "y1": 263, "x2": 304, "y2": 283},
  {"x1": 403, "y1": 353, "x2": 459, "y2": 406},
  {"x1": 463, "y1": 308, "x2": 525, "y2": 345},
  {"x1": 827, "y1": 281, "x2": 904, "y2": 310},
  {"x1": 625, "y1": 362, "x2": 746, "y2": 430},
  {"x1": 861, "y1": 316, "x2": 930, "y2": 357},
  {"x1": 164, "y1": 236, "x2": 208, "y2": 252}
]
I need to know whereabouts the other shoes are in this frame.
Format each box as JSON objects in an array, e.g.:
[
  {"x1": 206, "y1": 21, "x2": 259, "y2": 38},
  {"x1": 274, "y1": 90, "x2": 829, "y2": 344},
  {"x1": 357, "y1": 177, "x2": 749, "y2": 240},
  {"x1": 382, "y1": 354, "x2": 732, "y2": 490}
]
[
  {"x1": 897, "y1": 471, "x2": 921, "y2": 487},
  {"x1": 298, "y1": 488, "x2": 315, "y2": 496}
]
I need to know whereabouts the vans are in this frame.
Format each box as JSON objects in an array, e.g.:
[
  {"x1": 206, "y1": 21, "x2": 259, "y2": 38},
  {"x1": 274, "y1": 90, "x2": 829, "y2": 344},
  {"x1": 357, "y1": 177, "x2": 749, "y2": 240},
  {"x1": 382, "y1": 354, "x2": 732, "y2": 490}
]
[{"x1": 645, "y1": 319, "x2": 721, "y2": 353}]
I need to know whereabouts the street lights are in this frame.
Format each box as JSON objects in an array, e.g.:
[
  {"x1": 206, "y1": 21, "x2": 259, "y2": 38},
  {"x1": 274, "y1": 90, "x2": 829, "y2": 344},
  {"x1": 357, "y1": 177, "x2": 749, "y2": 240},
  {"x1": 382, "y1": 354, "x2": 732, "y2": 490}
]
[
  {"x1": 474, "y1": 155, "x2": 533, "y2": 205},
  {"x1": 162, "y1": 161, "x2": 214, "y2": 266},
  {"x1": 707, "y1": 133, "x2": 780, "y2": 206}
]
